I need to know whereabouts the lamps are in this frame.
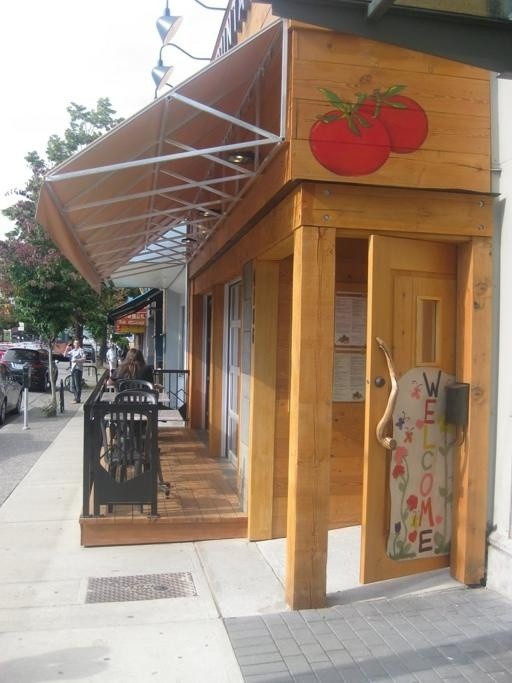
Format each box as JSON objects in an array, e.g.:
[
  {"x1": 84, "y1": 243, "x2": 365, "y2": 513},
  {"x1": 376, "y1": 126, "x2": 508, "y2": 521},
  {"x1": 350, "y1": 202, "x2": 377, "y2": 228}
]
[
  {"x1": 181, "y1": 236, "x2": 199, "y2": 244},
  {"x1": 227, "y1": 150, "x2": 264, "y2": 165},
  {"x1": 146, "y1": 46, "x2": 223, "y2": 98},
  {"x1": 196, "y1": 207, "x2": 222, "y2": 218},
  {"x1": 145, "y1": 2, "x2": 234, "y2": 44}
]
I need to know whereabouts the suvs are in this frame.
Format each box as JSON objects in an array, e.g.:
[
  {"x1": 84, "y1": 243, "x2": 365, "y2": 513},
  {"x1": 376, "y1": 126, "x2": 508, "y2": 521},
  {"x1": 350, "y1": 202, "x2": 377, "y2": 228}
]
[
  {"x1": 0, "y1": 345, "x2": 58, "y2": 391},
  {"x1": 83, "y1": 343, "x2": 95, "y2": 362}
]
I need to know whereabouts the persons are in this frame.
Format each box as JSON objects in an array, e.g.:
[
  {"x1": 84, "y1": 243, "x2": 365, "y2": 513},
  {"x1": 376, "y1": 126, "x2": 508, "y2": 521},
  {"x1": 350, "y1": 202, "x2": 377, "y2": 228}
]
[
  {"x1": 108, "y1": 348, "x2": 153, "y2": 392},
  {"x1": 104, "y1": 342, "x2": 121, "y2": 376},
  {"x1": 62, "y1": 339, "x2": 87, "y2": 404}
]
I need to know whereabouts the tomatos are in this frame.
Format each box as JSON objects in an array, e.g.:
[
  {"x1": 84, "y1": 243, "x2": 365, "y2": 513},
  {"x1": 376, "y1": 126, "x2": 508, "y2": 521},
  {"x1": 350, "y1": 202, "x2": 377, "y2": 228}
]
[{"x1": 304, "y1": 82, "x2": 428, "y2": 176}]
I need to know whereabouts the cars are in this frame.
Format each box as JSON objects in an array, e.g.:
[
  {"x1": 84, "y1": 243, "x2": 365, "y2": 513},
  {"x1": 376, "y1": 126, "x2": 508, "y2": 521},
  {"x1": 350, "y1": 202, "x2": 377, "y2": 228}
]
[
  {"x1": 0, "y1": 342, "x2": 14, "y2": 362},
  {"x1": 65, "y1": 343, "x2": 73, "y2": 359},
  {"x1": 0, "y1": 362, "x2": 23, "y2": 424}
]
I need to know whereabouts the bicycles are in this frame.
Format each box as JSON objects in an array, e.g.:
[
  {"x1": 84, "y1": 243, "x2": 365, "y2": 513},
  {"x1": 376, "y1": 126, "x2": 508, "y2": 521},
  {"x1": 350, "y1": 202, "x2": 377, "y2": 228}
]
[{"x1": 65, "y1": 365, "x2": 75, "y2": 392}]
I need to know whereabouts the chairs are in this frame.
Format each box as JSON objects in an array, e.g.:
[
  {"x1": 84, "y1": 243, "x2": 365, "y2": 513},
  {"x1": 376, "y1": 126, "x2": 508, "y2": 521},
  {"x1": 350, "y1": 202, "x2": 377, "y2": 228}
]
[{"x1": 103, "y1": 375, "x2": 173, "y2": 512}]
